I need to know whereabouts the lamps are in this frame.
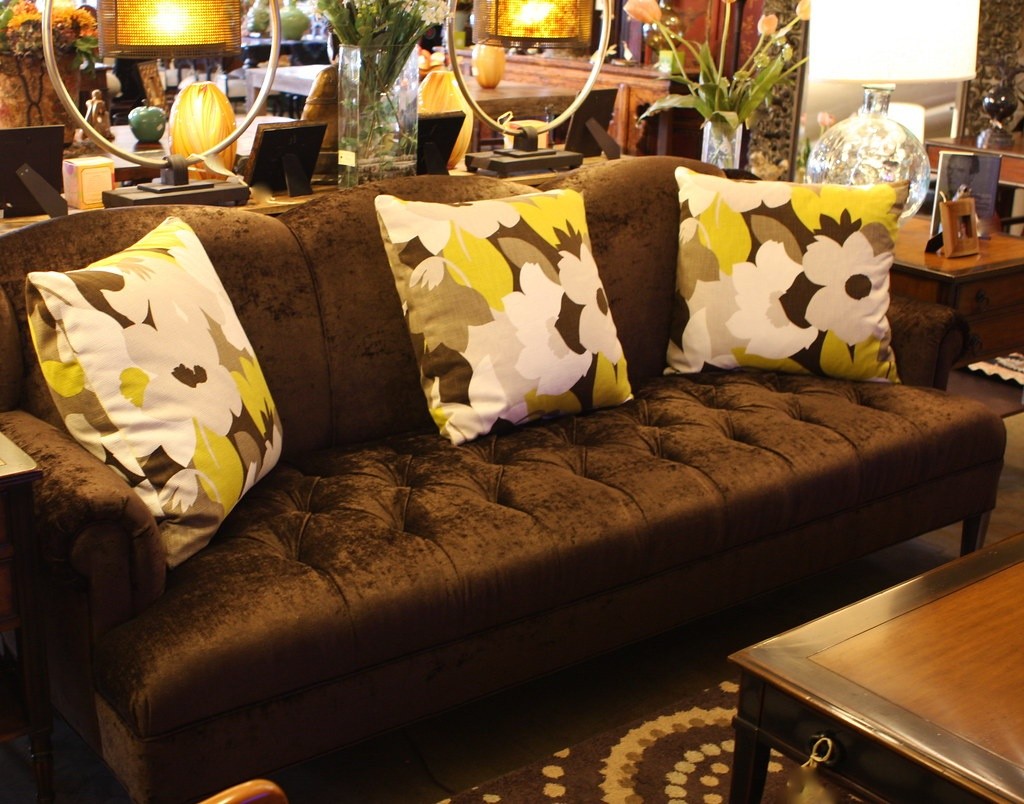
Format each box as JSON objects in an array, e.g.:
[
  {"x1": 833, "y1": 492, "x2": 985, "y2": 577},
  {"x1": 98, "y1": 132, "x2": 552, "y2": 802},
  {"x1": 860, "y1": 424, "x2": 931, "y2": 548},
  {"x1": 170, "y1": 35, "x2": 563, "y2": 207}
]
[
  {"x1": 169, "y1": 81, "x2": 239, "y2": 181},
  {"x1": 419, "y1": 70, "x2": 473, "y2": 169},
  {"x1": 43, "y1": 0, "x2": 283, "y2": 206},
  {"x1": 446, "y1": 0, "x2": 612, "y2": 178},
  {"x1": 471, "y1": 43, "x2": 505, "y2": 88}
]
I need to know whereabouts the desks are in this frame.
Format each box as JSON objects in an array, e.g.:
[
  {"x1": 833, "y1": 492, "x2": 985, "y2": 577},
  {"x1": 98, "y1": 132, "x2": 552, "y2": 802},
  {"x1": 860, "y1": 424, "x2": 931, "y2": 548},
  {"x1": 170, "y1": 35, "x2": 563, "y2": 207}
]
[
  {"x1": 246, "y1": 56, "x2": 618, "y2": 151},
  {"x1": 432, "y1": 47, "x2": 702, "y2": 159},
  {"x1": 0, "y1": 154, "x2": 630, "y2": 235}
]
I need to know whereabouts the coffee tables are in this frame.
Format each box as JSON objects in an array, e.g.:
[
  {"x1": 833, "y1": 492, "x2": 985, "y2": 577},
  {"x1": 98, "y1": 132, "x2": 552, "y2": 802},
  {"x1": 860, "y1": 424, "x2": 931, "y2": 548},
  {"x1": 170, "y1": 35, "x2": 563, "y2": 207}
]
[{"x1": 728, "y1": 531, "x2": 1024, "y2": 804}]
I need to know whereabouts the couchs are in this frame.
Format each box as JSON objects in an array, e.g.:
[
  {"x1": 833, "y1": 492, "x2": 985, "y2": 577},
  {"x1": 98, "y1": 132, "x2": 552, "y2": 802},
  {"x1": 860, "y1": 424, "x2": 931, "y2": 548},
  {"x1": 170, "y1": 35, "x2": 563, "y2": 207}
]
[{"x1": 0, "y1": 156, "x2": 1007, "y2": 804}]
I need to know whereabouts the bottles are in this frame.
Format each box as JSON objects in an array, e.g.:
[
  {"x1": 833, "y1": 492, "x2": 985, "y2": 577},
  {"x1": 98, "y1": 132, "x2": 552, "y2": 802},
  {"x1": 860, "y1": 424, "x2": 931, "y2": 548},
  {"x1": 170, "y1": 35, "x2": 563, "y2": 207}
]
[
  {"x1": 246, "y1": 0, "x2": 271, "y2": 32},
  {"x1": 279, "y1": 0, "x2": 309, "y2": 40},
  {"x1": 806, "y1": 82, "x2": 931, "y2": 224}
]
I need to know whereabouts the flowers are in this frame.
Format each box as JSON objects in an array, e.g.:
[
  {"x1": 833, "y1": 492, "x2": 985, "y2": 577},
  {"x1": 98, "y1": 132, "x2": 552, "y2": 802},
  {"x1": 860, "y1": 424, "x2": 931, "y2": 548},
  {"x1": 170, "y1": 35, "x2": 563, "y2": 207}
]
[
  {"x1": 0, "y1": 0, "x2": 104, "y2": 80},
  {"x1": 624, "y1": 0, "x2": 811, "y2": 164},
  {"x1": 310, "y1": 0, "x2": 452, "y2": 168}
]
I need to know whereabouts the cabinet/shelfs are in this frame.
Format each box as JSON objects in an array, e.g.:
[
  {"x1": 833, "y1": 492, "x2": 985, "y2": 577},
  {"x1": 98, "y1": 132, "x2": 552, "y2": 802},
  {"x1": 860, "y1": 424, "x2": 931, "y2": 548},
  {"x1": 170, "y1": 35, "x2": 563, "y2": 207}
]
[
  {"x1": 889, "y1": 246, "x2": 1024, "y2": 372},
  {"x1": 923, "y1": 135, "x2": 1024, "y2": 235}
]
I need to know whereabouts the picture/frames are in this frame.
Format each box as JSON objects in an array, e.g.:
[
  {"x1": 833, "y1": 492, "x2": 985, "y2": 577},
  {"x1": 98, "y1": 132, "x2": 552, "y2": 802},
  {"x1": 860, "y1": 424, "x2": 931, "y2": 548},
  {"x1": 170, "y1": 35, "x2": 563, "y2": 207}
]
[
  {"x1": 417, "y1": 111, "x2": 465, "y2": 175},
  {"x1": 939, "y1": 198, "x2": 980, "y2": 258},
  {"x1": 564, "y1": 88, "x2": 617, "y2": 156},
  {"x1": 244, "y1": 119, "x2": 328, "y2": 191},
  {"x1": 0, "y1": 125, "x2": 65, "y2": 218},
  {"x1": 135, "y1": 59, "x2": 167, "y2": 111}
]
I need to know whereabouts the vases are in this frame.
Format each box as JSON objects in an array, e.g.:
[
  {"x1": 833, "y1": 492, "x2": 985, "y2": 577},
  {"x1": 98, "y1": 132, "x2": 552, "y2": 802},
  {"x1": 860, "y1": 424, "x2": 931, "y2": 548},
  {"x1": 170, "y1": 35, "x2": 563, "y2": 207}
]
[
  {"x1": 248, "y1": 0, "x2": 271, "y2": 34},
  {"x1": 805, "y1": 83, "x2": 932, "y2": 223},
  {"x1": 338, "y1": 44, "x2": 419, "y2": 187},
  {"x1": 279, "y1": 0, "x2": 309, "y2": 41},
  {"x1": 642, "y1": 0, "x2": 686, "y2": 54},
  {"x1": 700, "y1": 121, "x2": 742, "y2": 169},
  {"x1": 0, "y1": 52, "x2": 81, "y2": 147},
  {"x1": 975, "y1": 65, "x2": 1024, "y2": 150}
]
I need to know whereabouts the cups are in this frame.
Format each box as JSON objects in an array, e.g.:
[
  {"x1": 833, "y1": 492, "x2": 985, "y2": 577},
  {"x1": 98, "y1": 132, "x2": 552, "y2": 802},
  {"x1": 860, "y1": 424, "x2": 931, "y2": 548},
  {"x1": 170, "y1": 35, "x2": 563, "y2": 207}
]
[{"x1": 128, "y1": 99, "x2": 166, "y2": 141}]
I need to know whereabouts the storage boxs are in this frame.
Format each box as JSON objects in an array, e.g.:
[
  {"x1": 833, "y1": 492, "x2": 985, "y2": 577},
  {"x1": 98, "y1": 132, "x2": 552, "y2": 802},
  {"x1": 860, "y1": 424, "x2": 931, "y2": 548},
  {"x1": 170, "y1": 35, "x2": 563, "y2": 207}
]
[{"x1": 61, "y1": 156, "x2": 115, "y2": 211}]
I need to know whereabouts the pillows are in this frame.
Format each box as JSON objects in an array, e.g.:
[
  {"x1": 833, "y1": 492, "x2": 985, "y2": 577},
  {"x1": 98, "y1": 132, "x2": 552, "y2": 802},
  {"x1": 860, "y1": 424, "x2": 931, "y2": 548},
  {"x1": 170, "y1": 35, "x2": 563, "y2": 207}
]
[
  {"x1": 663, "y1": 165, "x2": 910, "y2": 384},
  {"x1": 374, "y1": 188, "x2": 637, "y2": 448},
  {"x1": 25, "y1": 216, "x2": 284, "y2": 568}
]
